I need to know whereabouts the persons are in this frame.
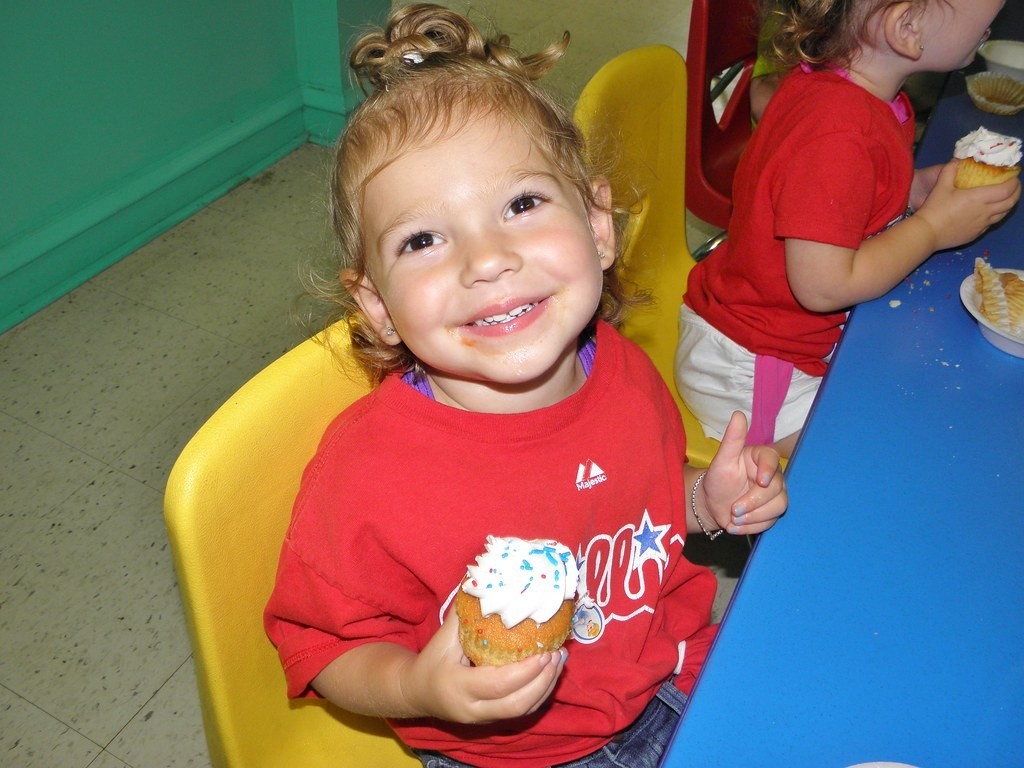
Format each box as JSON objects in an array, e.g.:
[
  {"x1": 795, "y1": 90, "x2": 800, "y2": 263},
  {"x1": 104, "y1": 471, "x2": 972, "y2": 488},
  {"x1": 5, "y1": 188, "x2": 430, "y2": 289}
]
[
  {"x1": 675, "y1": 0, "x2": 1020, "y2": 547},
  {"x1": 262, "y1": 4, "x2": 788, "y2": 768}
]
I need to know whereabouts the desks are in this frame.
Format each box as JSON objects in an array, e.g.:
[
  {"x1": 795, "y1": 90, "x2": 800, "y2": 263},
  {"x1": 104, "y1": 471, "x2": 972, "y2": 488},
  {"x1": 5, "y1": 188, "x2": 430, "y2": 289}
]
[{"x1": 660, "y1": 9, "x2": 1024, "y2": 768}]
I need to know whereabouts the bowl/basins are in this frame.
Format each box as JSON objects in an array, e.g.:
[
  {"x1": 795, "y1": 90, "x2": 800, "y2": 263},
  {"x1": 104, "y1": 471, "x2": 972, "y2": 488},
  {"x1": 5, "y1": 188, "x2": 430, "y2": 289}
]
[
  {"x1": 960, "y1": 268, "x2": 1024, "y2": 358},
  {"x1": 977, "y1": 40, "x2": 1024, "y2": 82}
]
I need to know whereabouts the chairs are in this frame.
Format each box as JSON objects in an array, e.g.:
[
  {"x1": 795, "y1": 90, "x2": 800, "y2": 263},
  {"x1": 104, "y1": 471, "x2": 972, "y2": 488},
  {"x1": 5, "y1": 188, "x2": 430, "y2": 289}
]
[
  {"x1": 686, "y1": 0, "x2": 759, "y2": 227},
  {"x1": 574, "y1": 44, "x2": 791, "y2": 476},
  {"x1": 162, "y1": 313, "x2": 424, "y2": 768}
]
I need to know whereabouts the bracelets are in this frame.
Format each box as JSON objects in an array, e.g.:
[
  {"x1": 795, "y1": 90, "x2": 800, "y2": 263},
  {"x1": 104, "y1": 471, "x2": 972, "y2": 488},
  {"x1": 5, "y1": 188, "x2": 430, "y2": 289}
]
[{"x1": 692, "y1": 472, "x2": 724, "y2": 542}]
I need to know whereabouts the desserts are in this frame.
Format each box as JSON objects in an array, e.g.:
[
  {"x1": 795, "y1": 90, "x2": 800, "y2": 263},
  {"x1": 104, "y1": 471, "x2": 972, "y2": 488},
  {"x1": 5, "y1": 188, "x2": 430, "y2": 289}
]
[
  {"x1": 953, "y1": 125, "x2": 1022, "y2": 189},
  {"x1": 456, "y1": 537, "x2": 580, "y2": 665}
]
